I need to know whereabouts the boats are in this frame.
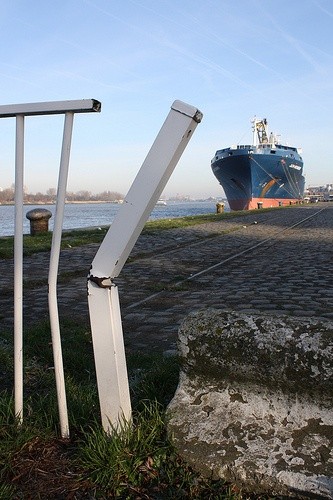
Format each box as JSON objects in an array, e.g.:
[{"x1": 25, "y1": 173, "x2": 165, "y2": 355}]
[{"x1": 209, "y1": 115, "x2": 307, "y2": 212}]
